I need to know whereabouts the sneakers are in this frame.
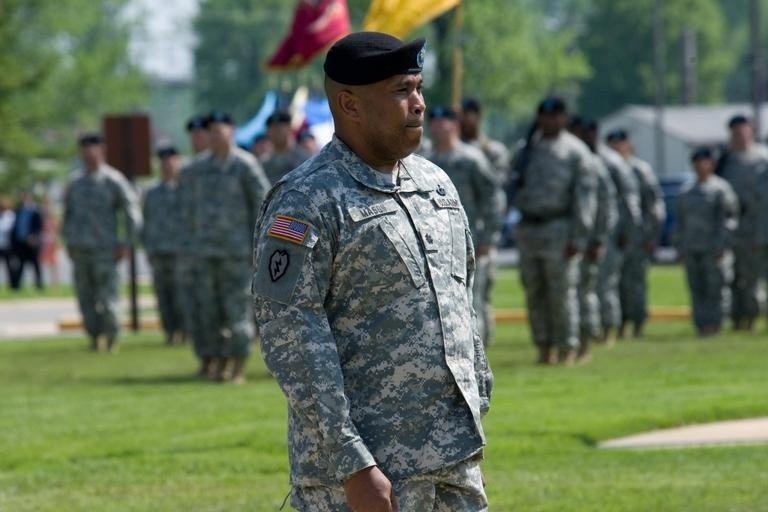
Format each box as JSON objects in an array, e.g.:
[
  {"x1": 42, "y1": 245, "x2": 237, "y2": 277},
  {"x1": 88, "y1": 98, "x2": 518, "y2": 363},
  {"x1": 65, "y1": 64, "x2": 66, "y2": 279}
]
[
  {"x1": 696, "y1": 317, "x2": 756, "y2": 337},
  {"x1": 87, "y1": 330, "x2": 118, "y2": 356}
]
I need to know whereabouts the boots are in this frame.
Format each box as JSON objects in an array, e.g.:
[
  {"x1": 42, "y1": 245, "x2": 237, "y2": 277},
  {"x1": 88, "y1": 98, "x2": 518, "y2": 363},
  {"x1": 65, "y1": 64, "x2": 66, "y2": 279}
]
[
  {"x1": 537, "y1": 318, "x2": 649, "y2": 365},
  {"x1": 164, "y1": 316, "x2": 248, "y2": 385}
]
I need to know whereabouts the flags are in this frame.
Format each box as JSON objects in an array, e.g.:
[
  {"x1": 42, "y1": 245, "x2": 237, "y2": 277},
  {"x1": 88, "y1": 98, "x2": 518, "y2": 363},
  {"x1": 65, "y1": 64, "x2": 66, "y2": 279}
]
[
  {"x1": 360, "y1": 0, "x2": 461, "y2": 42},
  {"x1": 267, "y1": 1, "x2": 351, "y2": 72}
]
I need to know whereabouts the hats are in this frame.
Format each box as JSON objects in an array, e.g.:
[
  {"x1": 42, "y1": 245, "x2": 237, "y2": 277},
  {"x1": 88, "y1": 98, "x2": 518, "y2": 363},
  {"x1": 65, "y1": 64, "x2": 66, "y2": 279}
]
[{"x1": 323, "y1": 31, "x2": 426, "y2": 86}]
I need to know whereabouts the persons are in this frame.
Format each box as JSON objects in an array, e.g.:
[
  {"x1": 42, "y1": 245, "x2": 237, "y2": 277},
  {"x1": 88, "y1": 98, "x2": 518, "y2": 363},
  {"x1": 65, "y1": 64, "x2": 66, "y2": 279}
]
[
  {"x1": 251, "y1": 32, "x2": 495, "y2": 512},
  {"x1": 424, "y1": 98, "x2": 767, "y2": 364},
  {"x1": 62, "y1": 110, "x2": 315, "y2": 384},
  {"x1": 1, "y1": 193, "x2": 58, "y2": 290}
]
